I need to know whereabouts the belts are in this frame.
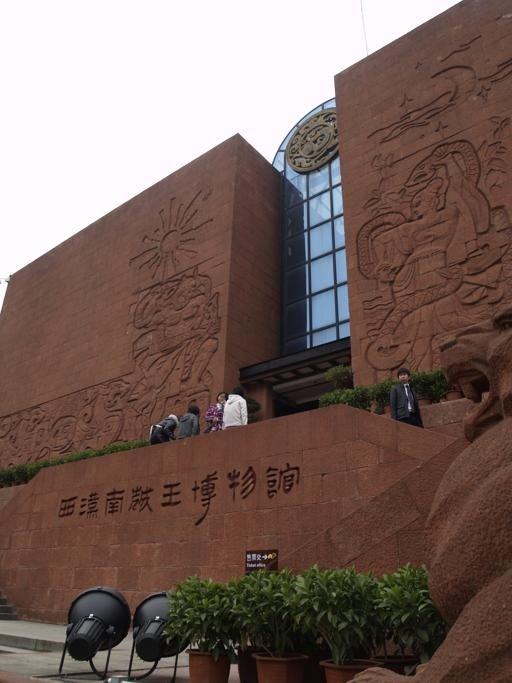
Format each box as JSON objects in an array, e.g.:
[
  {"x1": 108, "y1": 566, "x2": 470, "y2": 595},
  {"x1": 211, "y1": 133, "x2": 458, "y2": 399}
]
[{"x1": 409, "y1": 409, "x2": 417, "y2": 413}]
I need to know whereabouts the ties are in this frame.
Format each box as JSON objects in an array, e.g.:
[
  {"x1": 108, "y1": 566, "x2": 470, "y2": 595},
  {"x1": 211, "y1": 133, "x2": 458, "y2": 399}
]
[{"x1": 406, "y1": 384, "x2": 415, "y2": 408}]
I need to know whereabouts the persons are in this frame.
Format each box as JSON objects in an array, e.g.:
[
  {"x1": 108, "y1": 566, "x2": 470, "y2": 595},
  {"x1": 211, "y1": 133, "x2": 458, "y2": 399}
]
[
  {"x1": 390, "y1": 368, "x2": 423, "y2": 428},
  {"x1": 175, "y1": 405, "x2": 200, "y2": 439},
  {"x1": 221, "y1": 386, "x2": 248, "y2": 430},
  {"x1": 204, "y1": 392, "x2": 228, "y2": 433},
  {"x1": 150, "y1": 414, "x2": 179, "y2": 444}
]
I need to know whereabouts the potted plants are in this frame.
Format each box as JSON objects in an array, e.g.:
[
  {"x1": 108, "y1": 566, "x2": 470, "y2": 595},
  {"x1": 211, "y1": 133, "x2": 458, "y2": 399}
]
[
  {"x1": 160, "y1": 561, "x2": 450, "y2": 682},
  {"x1": 0, "y1": 462, "x2": 29, "y2": 488},
  {"x1": 372, "y1": 366, "x2": 461, "y2": 413}
]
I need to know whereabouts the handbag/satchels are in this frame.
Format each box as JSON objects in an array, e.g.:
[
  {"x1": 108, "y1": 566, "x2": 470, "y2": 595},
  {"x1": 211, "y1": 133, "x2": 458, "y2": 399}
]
[{"x1": 204, "y1": 423, "x2": 222, "y2": 433}]
